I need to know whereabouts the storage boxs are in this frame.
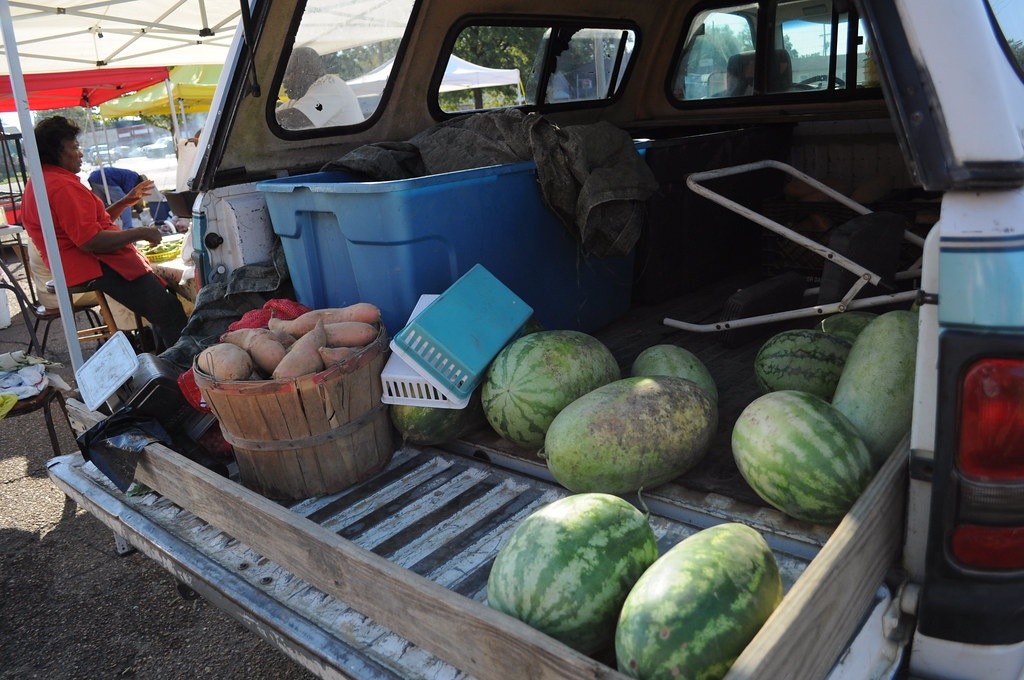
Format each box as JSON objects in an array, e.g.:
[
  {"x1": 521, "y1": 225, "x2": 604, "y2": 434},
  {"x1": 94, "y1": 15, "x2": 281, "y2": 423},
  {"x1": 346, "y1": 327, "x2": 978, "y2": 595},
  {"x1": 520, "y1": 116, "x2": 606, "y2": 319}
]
[
  {"x1": 637, "y1": 118, "x2": 799, "y2": 304},
  {"x1": 254, "y1": 136, "x2": 657, "y2": 354}
]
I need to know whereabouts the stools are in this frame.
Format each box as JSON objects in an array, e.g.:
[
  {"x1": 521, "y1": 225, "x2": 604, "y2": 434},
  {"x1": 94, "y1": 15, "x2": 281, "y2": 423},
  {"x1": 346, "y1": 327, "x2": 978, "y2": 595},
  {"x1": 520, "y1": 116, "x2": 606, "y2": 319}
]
[
  {"x1": 44, "y1": 279, "x2": 167, "y2": 358},
  {"x1": 8, "y1": 387, "x2": 81, "y2": 499}
]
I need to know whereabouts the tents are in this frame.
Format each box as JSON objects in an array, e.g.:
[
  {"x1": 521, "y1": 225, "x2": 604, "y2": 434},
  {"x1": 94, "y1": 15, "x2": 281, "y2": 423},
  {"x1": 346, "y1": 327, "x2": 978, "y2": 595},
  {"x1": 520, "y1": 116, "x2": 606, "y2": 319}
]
[{"x1": 0, "y1": 0, "x2": 528, "y2": 560}]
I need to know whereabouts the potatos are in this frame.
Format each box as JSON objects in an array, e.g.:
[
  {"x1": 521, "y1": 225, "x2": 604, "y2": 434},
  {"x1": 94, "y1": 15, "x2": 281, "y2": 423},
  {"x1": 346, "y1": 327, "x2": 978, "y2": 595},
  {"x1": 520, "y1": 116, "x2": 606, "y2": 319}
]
[{"x1": 198, "y1": 302, "x2": 381, "y2": 380}]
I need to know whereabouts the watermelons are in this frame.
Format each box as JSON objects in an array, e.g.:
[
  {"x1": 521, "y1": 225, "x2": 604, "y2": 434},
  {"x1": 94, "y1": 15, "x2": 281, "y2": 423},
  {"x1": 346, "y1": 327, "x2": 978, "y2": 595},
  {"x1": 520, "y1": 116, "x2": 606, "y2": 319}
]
[{"x1": 391, "y1": 311, "x2": 918, "y2": 680}]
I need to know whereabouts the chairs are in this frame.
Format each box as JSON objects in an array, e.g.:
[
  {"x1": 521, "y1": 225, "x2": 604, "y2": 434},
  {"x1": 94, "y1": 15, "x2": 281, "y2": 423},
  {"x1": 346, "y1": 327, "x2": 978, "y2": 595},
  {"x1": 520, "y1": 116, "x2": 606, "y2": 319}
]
[
  {"x1": 0, "y1": 257, "x2": 108, "y2": 358},
  {"x1": 701, "y1": 49, "x2": 814, "y2": 99},
  {"x1": 662, "y1": 159, "x2": 927, "y2": 335}
]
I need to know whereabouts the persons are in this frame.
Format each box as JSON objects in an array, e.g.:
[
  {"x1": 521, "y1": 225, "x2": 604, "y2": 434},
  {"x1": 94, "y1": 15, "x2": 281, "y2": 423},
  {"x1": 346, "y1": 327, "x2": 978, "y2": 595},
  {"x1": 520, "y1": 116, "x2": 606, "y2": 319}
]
[
  {"x1": 187, "y1": 47, "x2": 366, "y2": 146},
  {"x1": 88, "y1": 166, "x2": 147, "y2": 248},
  {"x1": 21, "y1": 114, "x2": 190, "y2": 357}
]
